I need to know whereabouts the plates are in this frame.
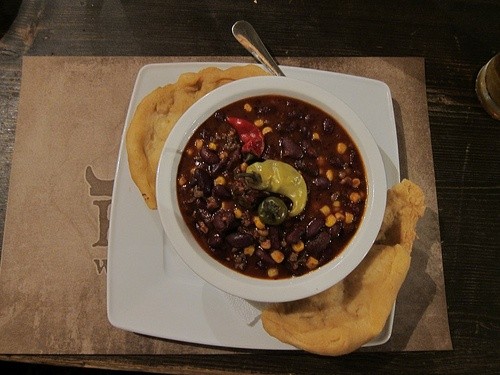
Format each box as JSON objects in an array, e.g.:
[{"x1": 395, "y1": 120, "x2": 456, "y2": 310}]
[{"x1": 106, "y1": 61, "x2": 401, "y2": 349}]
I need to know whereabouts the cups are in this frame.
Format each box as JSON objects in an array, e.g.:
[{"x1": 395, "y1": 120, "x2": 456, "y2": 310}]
[{"x1": 475, "y1": 52, "x2": 500, "y2": 121}]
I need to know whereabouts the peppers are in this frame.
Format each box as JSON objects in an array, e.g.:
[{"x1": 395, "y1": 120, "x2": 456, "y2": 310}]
[{"x1": 226, "y1": 116, "x2": 308, "y2": 225}]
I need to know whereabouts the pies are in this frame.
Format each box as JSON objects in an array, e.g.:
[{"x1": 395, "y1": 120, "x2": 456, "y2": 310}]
[
  {"x1": 263, "y1": 178, "x2": 426, "y2": 356},
  {"x1": 126, "y1": 65, "x2": 271, "y2": 210}
]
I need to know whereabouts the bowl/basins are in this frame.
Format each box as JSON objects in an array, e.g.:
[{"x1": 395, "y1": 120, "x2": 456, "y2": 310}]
[{"x1": 155, "y1": 75, "x2": 386, "y2": 303}]
